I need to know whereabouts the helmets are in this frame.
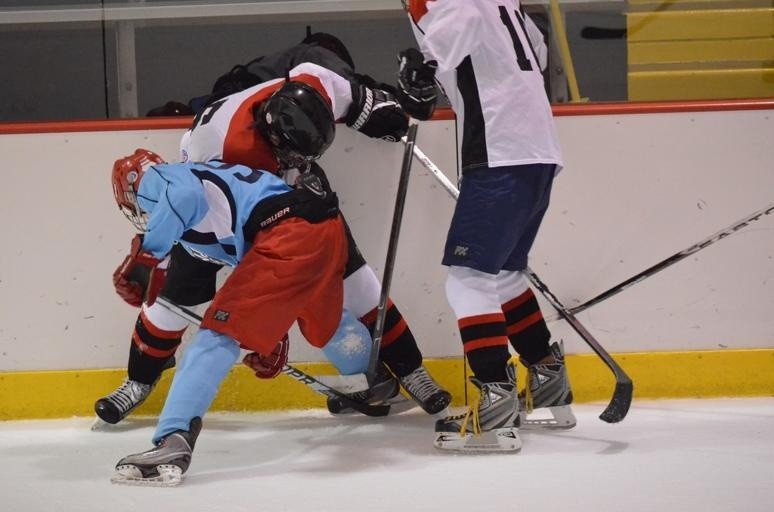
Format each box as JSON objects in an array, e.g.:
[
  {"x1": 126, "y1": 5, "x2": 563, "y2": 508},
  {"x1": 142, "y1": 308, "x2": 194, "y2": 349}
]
[
  {"x1": 111, "y1": 148, "x2": 166, "y2": 233},
  {"x1": 256, "y1": 79, "x2": 335, "y2": 166}
]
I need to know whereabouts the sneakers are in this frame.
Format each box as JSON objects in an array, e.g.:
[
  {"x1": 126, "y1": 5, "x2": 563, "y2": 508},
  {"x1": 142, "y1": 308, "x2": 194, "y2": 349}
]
[
  {"x1": 518, "y1": 338, "x2": 574, "y2": 410},
  {"x1": 93, "y1": 376, "x2": 151, "y2": 424},
  {"x1": 115, "y1": 414, "x2": 202, "y2": 479},
  {"x1": 327, "y1": 368, "x2": 398, "y2": 414},
  {"x1": 399, "y1": 365, "x2": 452, "y2": 414},
  {"x1": 434, "y1": 362, "x2": 520, "y2": 430}
]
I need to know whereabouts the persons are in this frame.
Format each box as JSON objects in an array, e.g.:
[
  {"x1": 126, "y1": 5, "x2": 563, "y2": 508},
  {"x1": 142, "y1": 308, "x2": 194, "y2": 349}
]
[
  {"x1": 111, "y1": 147, "x2": 391, "y2": 480},
  {"x1": 403, "y1": 0, "x2": 576, "y2": 435},
  {"x1": 148, "y1": 19, "x2": 398, "y2": 116},
  {"x1": 93, "y1": 61, "x2": 452, "y2": 425}
]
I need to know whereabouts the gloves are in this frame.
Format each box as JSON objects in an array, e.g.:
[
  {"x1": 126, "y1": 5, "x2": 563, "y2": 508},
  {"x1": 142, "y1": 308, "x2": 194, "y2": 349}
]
[
  {"x1": 394, "y1": 48, "x2": 437, "y2": 120},
  {"x1": 239, "y1": 332, "x2": 290, "y2": 378},
  {"x1": 112, "y1": 234, "x2": 171, "y2": 307},
  {"x1": 344, "y1": 79, "x2": 408, "y2": 143}
]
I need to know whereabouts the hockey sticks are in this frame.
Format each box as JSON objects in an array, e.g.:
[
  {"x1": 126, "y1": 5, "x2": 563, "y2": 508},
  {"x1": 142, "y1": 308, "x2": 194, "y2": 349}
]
[
  {"x1": 149, "y1": 295, "x2": 390, "y2": 416},
  {"x1": 402, "y1": 137, "x2": 633, "y2": 424},
  {"x1": 311, "y1": 116, "x2": 419, "y2": 393}
]
